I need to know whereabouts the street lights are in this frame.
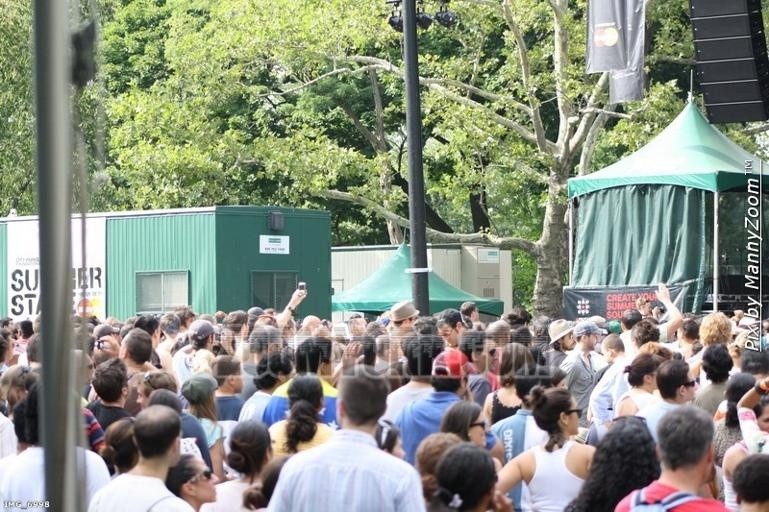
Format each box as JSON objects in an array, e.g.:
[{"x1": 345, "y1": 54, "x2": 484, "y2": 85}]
[{"x1": 384, "y1": 1, "x2": 458, "y2": 312}]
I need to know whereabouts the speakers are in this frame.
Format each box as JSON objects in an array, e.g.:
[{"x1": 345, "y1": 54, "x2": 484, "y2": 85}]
[{"x1": 689, "y1": 1, "x2": 769, "y2": 125}]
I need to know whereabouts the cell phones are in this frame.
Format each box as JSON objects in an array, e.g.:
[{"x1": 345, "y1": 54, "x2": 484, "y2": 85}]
[{"x1": 298, "y1": 282, "x2": 306, "y2": 291}]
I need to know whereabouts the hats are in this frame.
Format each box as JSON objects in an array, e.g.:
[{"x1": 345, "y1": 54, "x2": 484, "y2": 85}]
[
  {"x1": 187, "y1": 320, "x2": 219, "y2": 340},
  {"x1": 181, "y1": 372, "x2": 218, "y2": 403},
  {"x1": 431, "y1": 348, "x2": 475, "y2": 377},
  {"x1": 387, "y1": 300, "x2": 421, "y2": 322},
  {"x1": 547, "y1": 319, "x2": 573, "y2": 344},
  {"x1": 93, "y1": 324, "x2": 119, "y2": 339},
  {"x1": 573, "y1": 314, "x2": 623, "y2": 339}
]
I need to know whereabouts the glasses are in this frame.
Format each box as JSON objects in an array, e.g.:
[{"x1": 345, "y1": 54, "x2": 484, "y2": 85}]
[
  {"x1": 184, "y1": 467, "x2": 212, "y2": 486},
  {"x1": 566, "y1": 407, "x2": 584, "y2": 418}
]
